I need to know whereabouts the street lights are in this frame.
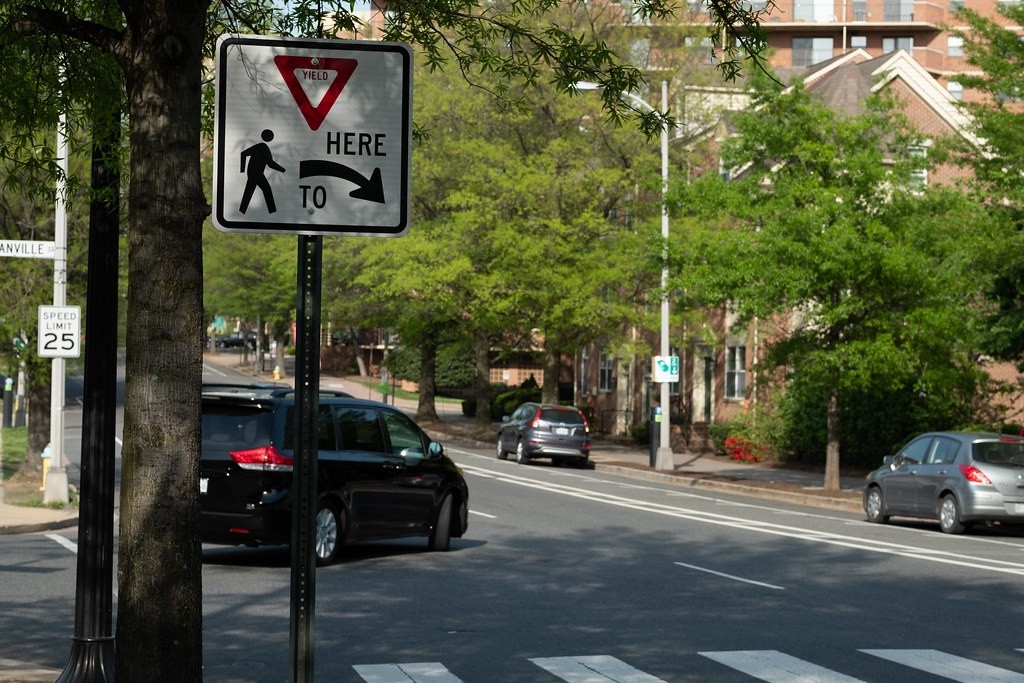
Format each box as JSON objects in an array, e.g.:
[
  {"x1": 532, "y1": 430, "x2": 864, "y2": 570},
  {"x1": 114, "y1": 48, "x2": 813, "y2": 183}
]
[{"x1": 565, "y1": 73, "x2": 682, "y2": 473}]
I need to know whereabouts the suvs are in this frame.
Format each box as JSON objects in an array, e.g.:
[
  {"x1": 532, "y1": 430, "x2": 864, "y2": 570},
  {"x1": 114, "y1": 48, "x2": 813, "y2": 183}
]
[
  {"x1": 498, "y1": 402, "x2": 594, "y2": 466},
  {"x1": 192, "y1": 383, "x2": 471, "y2": 564}
]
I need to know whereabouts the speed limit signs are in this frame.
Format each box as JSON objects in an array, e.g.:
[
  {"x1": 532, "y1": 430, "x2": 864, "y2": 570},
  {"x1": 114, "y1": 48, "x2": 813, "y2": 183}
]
[{"x1": 36, "y1": 304, "x2": 80, "y2": 358}]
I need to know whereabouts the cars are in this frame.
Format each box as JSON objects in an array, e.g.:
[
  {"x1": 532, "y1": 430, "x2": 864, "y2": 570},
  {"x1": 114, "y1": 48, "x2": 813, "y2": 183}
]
[
  {"x1": 218, "y1": 330, "x2": 258, "y2": 351},
  {"x1": 861, "y1": 435, "x2": 1024, "y2": 536}
]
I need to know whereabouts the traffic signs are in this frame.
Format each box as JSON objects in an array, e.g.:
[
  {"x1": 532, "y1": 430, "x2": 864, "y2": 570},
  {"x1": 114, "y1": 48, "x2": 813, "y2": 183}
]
[{"x1": 217, "y1": 33, "x2": 416, "y2": 241}]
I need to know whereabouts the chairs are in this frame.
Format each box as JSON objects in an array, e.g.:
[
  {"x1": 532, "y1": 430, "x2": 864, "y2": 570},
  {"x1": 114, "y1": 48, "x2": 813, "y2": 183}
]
[{"x1": 987, "y1": 450, "x2": 1003, "y2": 461}]
